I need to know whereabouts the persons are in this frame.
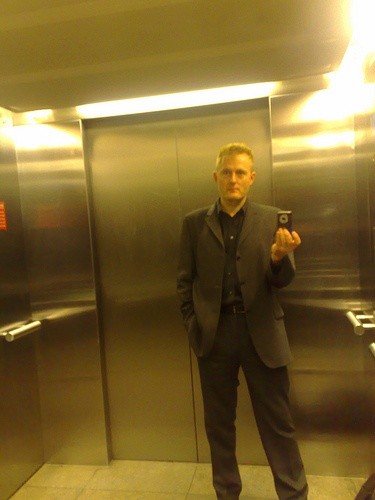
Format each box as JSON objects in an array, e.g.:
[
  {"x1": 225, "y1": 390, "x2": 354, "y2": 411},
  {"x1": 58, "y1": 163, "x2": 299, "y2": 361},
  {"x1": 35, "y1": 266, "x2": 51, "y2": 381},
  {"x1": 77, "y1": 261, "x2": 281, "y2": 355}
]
[{"x1": 175, "y1": 143, "x2": 309, "y2": 500}]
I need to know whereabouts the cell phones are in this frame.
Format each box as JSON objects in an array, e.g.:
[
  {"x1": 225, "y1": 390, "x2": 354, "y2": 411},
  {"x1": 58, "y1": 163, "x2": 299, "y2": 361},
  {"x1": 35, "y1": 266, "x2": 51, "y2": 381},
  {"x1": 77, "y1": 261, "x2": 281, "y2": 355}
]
[{"x1": 277, "y1": 210, "x2": 292, "y2": 234}]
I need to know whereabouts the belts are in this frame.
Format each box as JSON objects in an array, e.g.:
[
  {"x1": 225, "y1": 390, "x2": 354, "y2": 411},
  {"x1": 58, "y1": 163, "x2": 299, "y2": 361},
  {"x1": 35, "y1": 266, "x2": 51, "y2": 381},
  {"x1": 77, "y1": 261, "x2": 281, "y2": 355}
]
[{"x1": 220, "y1": 304, "x2": 246, "y2": 315}]
